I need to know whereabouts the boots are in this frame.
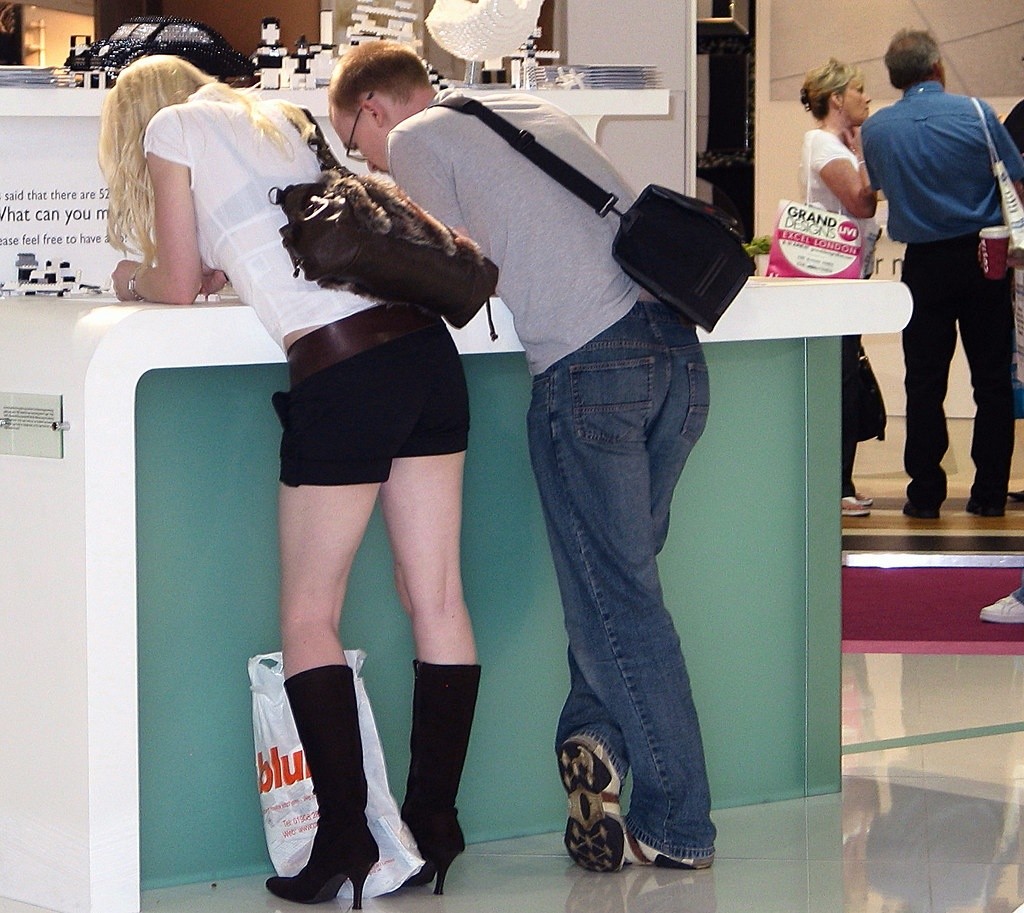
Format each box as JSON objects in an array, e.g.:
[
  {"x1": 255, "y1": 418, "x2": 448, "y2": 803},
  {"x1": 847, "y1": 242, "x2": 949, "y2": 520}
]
[
  {"x1": 399, "y1": 659, "x2": 482, "y2": 895},
  {"x1": 264, "y1": 664, "x2": 380, "y2": 910}
]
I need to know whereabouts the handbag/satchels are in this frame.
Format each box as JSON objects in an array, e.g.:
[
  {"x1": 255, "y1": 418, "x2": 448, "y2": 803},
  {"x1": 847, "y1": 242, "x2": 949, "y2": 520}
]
[
  {"x1": 972, "y1": 97, "x2": 1024, "y2": 252},
  {"x1": 427, "y1": 95, "x2": 758, "y2": 335},
  {"x1": 266, "y1": 105, "x2": 501, "y2": 343},
  {"x1": 248, "y1": 648, "x2": 425, "y2": 901},
  {"x1": 766, "y1": 198, "x2": 867, "y2": 279}
]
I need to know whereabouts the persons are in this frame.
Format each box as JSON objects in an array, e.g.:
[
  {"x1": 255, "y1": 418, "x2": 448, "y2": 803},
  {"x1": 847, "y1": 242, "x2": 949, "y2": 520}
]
[
  {"x1": 859, "y1": 26, "x2": 1024, "y2": 519},
  {"x1": 1000, "y1": 101, "x2": 1024, "y2": 502},
  {"x1": 103, "y1": 57, "x2": 480, "y2": 906},
  {"x1": 328, "y1": 40, "x2": 718, "y2": 872},
  {"x1": 980, "y1": 585, "x2": 1024, "y2": 624},
  {"x1": 800, "y1": 57, "x2": 886, "y2": 517}
]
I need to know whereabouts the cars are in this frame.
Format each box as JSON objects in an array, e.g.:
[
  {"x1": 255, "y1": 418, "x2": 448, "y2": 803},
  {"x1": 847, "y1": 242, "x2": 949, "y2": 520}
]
[{"x1": 64, "y1": 15, "x2": 256, "y2": 88}]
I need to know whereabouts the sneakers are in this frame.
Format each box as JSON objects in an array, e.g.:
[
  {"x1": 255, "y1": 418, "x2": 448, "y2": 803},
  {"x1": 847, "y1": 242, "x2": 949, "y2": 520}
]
[
  {"x1": 619, "y1": 813, "x2": 714, "y2": 871},
  {"x1": 979, "y1": 595, "x2": 1023, "y2": 623},
  {"x1": 557, "y1": 735, "x2": 626, "y2": 871}
]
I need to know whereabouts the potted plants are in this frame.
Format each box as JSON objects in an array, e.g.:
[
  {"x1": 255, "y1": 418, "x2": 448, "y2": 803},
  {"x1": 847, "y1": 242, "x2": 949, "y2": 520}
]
[{"x1": 743, "y1": 235, "x2": 771, "y2": 278}]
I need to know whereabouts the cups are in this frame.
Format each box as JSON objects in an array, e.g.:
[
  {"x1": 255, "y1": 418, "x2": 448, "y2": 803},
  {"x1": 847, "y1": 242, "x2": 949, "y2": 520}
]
[{"x1": 978, "y1": 226, "x2": 1010, "y2": 280}]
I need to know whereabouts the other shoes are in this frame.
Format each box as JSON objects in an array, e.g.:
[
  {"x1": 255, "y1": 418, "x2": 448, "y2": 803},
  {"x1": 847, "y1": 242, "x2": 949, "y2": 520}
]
[
  {"x1": 841, "y1": 489, "x2": 874, "y2": 516},
  {"x1": 903, "y1": 501, "x2": 941, "y2": 518},
  {"x1": 965, "y1": 500, "x2": 1006, "y2": 516},
  {"x1": 1009, "y1": 490, "x2": 1024, "y2": 498}
]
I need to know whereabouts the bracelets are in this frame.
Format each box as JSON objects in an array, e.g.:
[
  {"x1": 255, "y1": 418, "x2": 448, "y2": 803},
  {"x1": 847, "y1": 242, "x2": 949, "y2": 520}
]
[
  {"x1": 858, "y1": 158, "x2": 866, "y2": 165},
  {"x1": 128, "y1": 264, "x2": 145, "y2": 304}
]
